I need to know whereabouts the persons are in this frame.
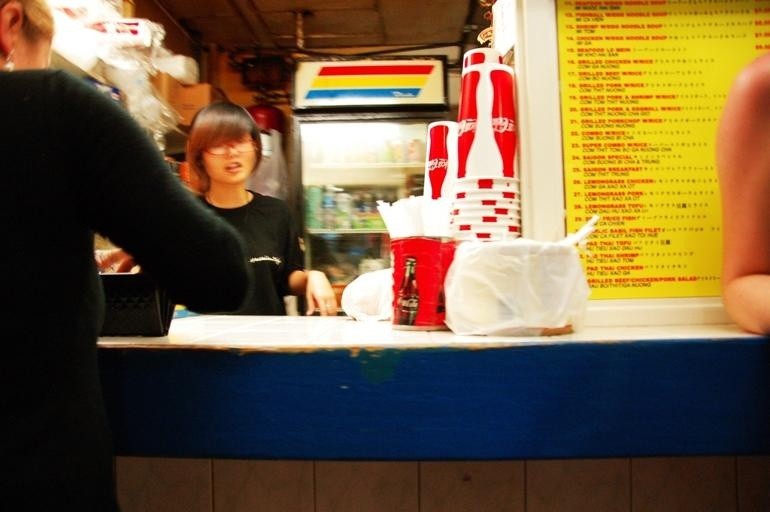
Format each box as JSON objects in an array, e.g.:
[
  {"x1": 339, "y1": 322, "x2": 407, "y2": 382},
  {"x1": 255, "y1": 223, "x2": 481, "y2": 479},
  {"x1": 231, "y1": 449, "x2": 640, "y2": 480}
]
[
  {"x1": 716, "y1": 49, "x2": 769, "y2": 335},
  {"x1": 184, "y1": 100, "x2": 340, "y2": 322},
  {"x1": 0, "y1": 0, "x2": 254, "y2": 512}
]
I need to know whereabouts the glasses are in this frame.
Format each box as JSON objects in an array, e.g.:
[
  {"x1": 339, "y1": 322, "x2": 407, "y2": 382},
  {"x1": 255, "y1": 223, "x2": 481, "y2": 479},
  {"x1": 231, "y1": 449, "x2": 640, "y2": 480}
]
[{"x1": 206, "y1": 140, "x2": 256, "y2": 156}]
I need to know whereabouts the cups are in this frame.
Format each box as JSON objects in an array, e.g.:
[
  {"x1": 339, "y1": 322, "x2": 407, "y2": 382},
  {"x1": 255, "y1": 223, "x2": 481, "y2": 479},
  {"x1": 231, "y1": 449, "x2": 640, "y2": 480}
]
[{"x1": 422, "y1": 47, "x2": 521, "y2": 242}]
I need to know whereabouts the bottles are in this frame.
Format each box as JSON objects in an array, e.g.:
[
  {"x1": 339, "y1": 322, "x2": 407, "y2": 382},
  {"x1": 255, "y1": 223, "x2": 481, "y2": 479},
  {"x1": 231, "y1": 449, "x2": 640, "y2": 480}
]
[
  {"x1": 396, "y1": 258, "x2": 419, "y2": 326},
  {"x1": 306, "y1": 183, "x2": 396, "y2": 229}
]
